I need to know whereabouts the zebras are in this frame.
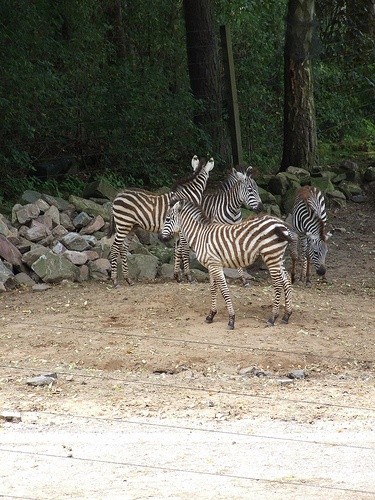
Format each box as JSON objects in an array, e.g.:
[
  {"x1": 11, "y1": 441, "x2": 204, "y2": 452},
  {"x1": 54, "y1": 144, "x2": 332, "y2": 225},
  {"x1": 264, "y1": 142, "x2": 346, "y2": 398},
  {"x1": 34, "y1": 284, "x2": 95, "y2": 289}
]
[
  {"x1": 105, "y1": 150, "x2": 263, "y2": 287},
  {"x1": 160, "y1": 196, "x2": 300, "y2": 332},
  {"x1": 284, "y1": 185, "x2": 335, "y2": 289}
]
